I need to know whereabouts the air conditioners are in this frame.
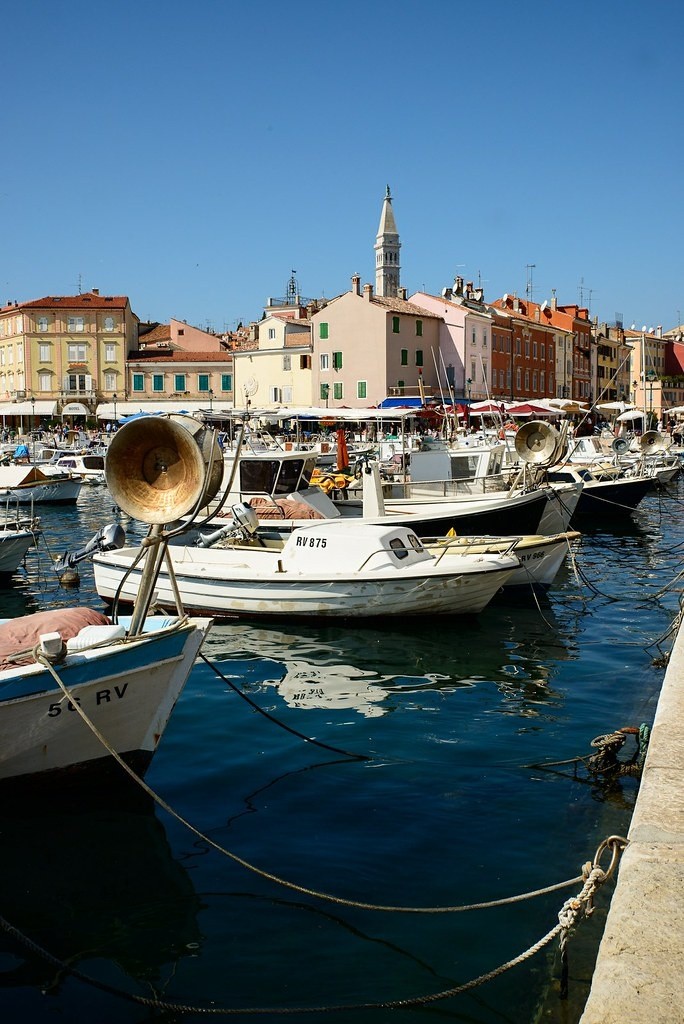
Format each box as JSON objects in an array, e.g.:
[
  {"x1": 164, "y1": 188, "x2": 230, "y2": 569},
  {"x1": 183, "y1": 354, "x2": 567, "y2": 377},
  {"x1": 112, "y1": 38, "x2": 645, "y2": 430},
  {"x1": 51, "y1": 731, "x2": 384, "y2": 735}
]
[
  {"x1": 390, "y1": 389, "x2": 400, "y2": 395},
  {"x1": 161, "y1": 342, "x2": 165, "y2": 346},
  {"x1": 156, "y1": 342, "x2": 160, "y2": 346},
  {"x1": 167, "y1": 341, "x2": 173, "y2": 346},
  {"x1": 141, "y1": 344, "x2": 146, "y2": 348}
]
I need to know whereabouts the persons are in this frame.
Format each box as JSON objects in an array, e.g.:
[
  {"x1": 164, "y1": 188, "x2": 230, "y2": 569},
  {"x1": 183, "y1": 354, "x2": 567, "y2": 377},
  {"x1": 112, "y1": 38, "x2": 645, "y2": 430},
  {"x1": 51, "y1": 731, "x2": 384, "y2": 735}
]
[
  {"x1": 552, "y1": 418, "x2": 561, "y2": 433},
  {"x1": 655, "y1": 418, "x2": 663, "y2": 435},
  {"x1": 0, "y1": 422, "x2": 119, "y2": 441},
  {"x1": 215, "y1": 427, "x2": 225, "y2": 453},
  {"x1": 244, "y1": 422, "x2": 252, "y2": 441},
  {"x1": 667, "y1": 417, "x2": 683, "y2": 447},
  {"x1": 292, "y1": 422, "x2": 438, "y2": 442},
  {"x1": 469, "y1": 425, "x2": 477, "y2": 434},
  {"x1": 336, "y1": 397, "x2": 591, "y2": 435},
  {"x1": 566, "y1": 421, "x2": 574, "y2": 434},
  {"x1": 613, "y1": 420, "x2": 619, "y2": 437}
]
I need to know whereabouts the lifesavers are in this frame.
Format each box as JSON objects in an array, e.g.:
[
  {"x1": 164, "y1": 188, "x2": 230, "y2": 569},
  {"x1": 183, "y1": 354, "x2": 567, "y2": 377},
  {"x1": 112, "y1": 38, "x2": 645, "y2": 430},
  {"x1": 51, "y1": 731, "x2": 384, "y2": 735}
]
[{"x1": 500, "y1": 424, "x2": 520, "y2": 439}]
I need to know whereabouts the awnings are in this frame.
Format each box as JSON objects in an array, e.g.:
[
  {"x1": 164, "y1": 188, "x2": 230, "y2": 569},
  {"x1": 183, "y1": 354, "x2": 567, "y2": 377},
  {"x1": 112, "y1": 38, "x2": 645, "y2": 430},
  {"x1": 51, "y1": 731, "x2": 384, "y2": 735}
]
[
  {"x1": 0, "y1": 400, "x2": 58, "y2": 416},
  {"x1": 377, "y1": 399, "x2": 430, "y2": 407},
  {"x1": 94, "y1": 402, "x2": 233, "y2": 421},
  {"x1": 441, "y1": 400, "x2": 474, "y2": 405}
]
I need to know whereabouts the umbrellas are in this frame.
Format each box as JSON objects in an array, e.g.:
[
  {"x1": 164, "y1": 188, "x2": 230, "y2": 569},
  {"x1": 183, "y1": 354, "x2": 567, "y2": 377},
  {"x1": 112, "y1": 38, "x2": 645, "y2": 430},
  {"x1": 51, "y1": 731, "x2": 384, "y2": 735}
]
[
  {"x1": 336, "y1": 427, "x2": 349, "y2": 470},
  {"x1": 615, "y1": 409, "x2": 645, "y2": 435},
  {"x1": 663, "y1": 405, "x2": 683, "y2": 414},
  {"x1": 97, "y1": 413, "x2": 126, "y2": 432},
  {"x1": 595, "y1": 401, "x2": 636, "y2": 438}
]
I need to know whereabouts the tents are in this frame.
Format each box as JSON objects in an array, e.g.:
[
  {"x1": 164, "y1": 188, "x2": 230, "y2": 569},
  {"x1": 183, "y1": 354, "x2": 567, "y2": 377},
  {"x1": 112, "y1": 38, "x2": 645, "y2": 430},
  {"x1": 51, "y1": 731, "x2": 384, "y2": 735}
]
[{"x1": 117, "y1": 409, "x2": 189, "y2": 432}]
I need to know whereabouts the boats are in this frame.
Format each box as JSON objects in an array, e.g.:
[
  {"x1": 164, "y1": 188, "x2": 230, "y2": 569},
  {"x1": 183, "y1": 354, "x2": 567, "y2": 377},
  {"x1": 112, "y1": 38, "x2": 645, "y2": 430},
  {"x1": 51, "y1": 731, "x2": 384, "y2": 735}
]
[
  {"x1": 87, "y1": 500, "x2": 523, "y2": 623},
  {"x1": 0, "y1": 463, "x2": 86, "y2": 506},
  {"x1": 0, "y1": 492, "x2": 42, "y2": 573},
  {"x1": 0, "y1": 346, "x2": 684, "y2": 544},
  {"x1": 0, "y1": 411, "x2": 225, "y2": 804},
  {"x1": 179, "y1": 398, "x2": 584, "y2": 541},
  {"x1": 208, "y1": 524, "x2": 583, "y2": 604}
]
